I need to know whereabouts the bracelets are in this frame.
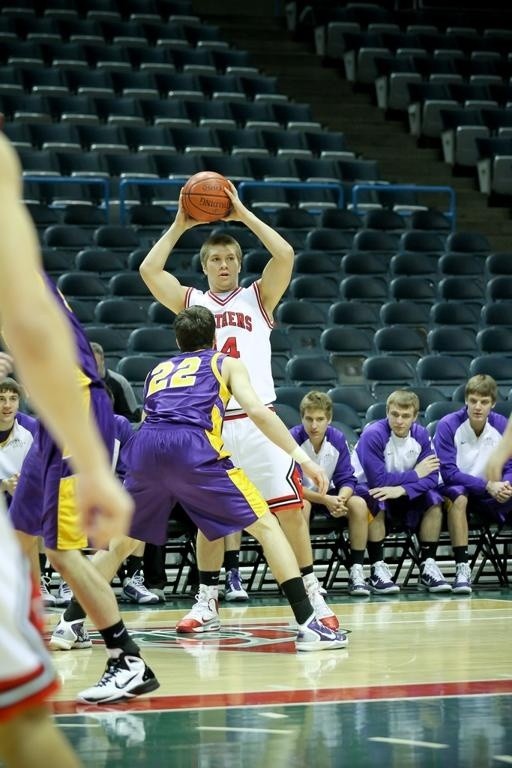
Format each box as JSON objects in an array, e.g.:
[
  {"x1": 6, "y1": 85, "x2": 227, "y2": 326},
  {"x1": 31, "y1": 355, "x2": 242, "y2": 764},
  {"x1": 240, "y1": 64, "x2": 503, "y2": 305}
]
[{"x1": 286, "y1": 446, "x2": 311, "y2": 467}]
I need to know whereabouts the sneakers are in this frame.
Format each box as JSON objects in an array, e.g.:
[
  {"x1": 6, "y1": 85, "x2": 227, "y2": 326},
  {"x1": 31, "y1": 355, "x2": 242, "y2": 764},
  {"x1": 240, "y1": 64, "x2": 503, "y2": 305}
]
[
  {"x1": 50, "y1": 612, "x2": 94, "y2": 650},
  {"x1": 77, "y1": 648, "x2": 159, "y2": 705},
  {"x1": 451, "y1": 561, "x2": 473, "y2": 593},
  {"x1": 306, "y1": 581, "x2": 341, "y2": 631},
  {"x1": 367, "y1": 560, "x2": 400, "y2": 594},
  {"x1": 177, "y1": 587, "x2": 223, "y2": 632},
  {"x1": 226, "y1": 568, "x2": 249, "y2": 601},
  {"x1": 38, "y1": 574, "x2": 74, "y2": 607},
  {"x1": 293, "y1": 611, "x2": 348, "y2": 650},
  {"x1": 348, "y1": 564, "x2": 372, "y2": 596},
  {"x1": 415, "y1": 556, "x2": 452, "y2": 593},
  {"x1": 119, "y1": 569, "x2": 167, "y2": 603}
]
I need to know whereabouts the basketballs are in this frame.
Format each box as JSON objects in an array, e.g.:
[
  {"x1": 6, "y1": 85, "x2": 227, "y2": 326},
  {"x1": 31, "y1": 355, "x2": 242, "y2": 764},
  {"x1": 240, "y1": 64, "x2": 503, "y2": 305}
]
[{"x1": 184, "y1": 172, "x2": 234, "y2": 223}]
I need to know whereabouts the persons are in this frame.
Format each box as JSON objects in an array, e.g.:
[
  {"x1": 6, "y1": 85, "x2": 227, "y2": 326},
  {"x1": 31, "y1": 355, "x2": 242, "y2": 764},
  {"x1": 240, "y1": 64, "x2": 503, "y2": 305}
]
[
  {"x1": 483, "y1": 411, "x2": 512, "y2": 482},
  {"x1": 39, "y1": 303, "x2": 351, "y2": 654},
  {"x1": 0, "y1": 351, "x2": 253, "y2": 609},
  {"x1": 431, "y1": 372, "x2": 512, "y2": 596},
  {"x1": 9, "y1": 265, "x2": 162, "y2": 712},
  {"x1": 352, "y1": 390, "x2": 453, "y2": 595},
  {"x1": 283, "y1": 389, "x2": 372, "y2": 598},
  {"x1": 0, "y1": 130, "x2": 135, "y2": 765},
  {"x1": 140, "y1": 178, "x2": 341, "y2": 635}
]
